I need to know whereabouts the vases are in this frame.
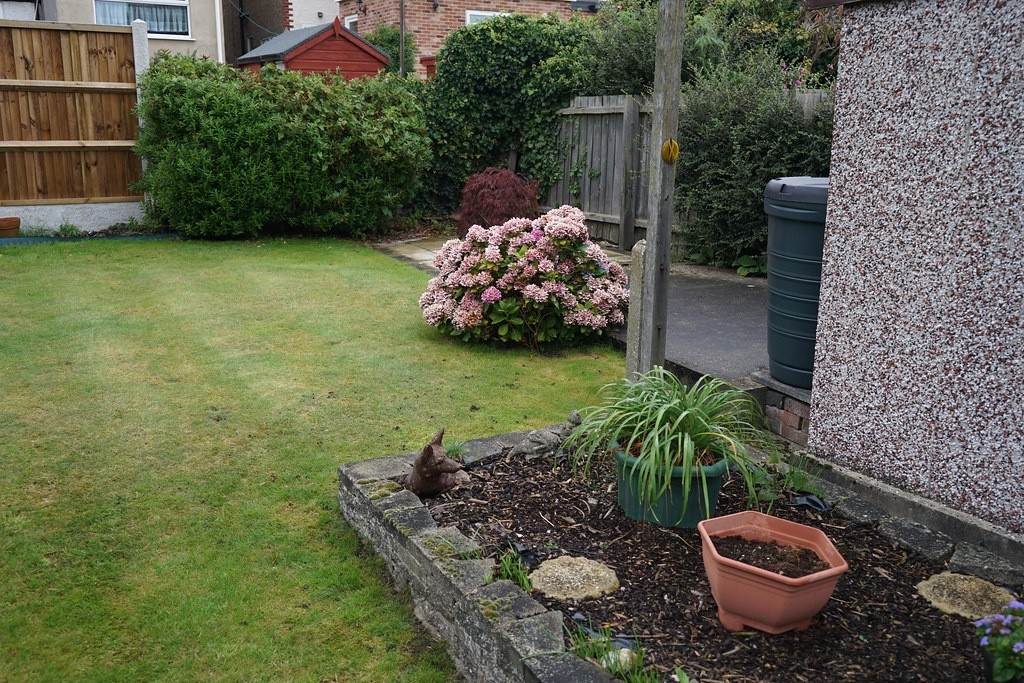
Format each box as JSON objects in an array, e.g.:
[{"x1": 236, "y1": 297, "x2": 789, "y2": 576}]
[
  {"x1": 0, "y1": 217, "x2": 21, "y2": 236},
  {"x1": 696, "y1": 511, "x2": 849, "y2": 637}
]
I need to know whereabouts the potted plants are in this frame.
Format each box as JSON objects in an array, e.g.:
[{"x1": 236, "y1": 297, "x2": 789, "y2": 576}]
[{"x1": 553, "y1": 366, "x2": 790, "y2": 531}]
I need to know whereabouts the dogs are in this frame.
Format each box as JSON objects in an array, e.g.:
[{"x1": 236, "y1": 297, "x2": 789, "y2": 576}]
[{"x1": 404, "y1": 428, "x2": 473, "y2": 498}]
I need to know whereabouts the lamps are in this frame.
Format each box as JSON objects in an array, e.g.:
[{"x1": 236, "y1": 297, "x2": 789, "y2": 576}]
[
  {"x1": 355, "y1": 0, "x2": 366, "y2": 16},
  {"x1": 433, "y1": 0, "x2": 440, "y2": 11}
]
